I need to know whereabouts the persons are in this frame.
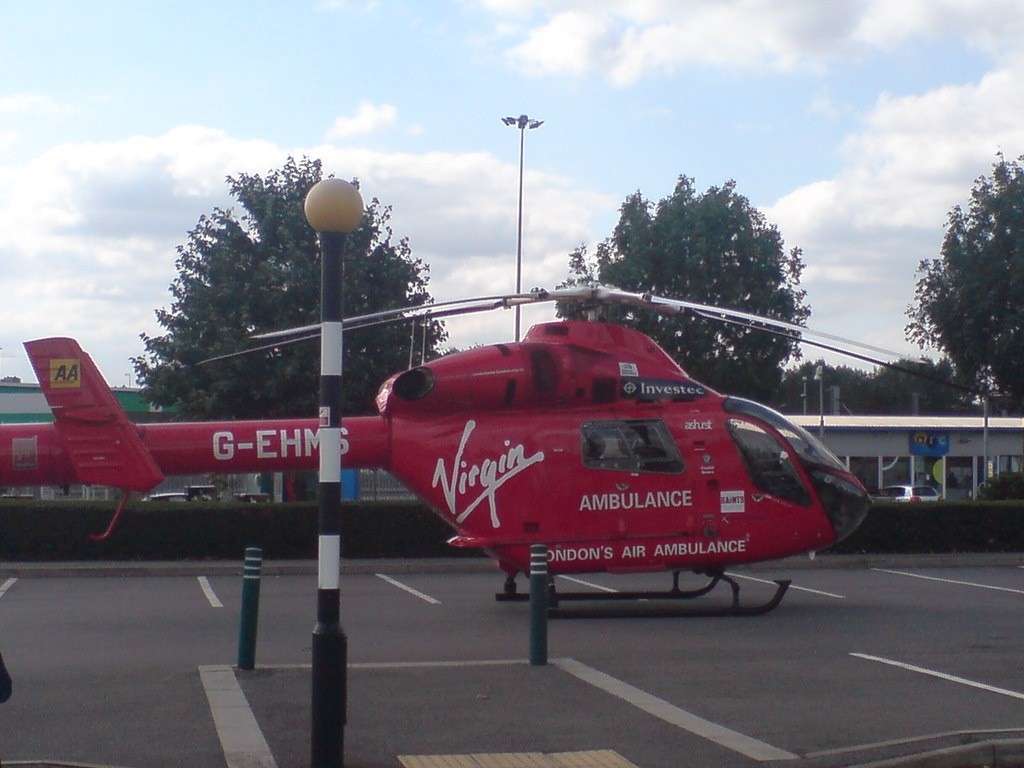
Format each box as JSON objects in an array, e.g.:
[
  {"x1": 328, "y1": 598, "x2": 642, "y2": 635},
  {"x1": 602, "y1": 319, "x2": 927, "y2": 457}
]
[
  {"x1": 735, "y1": 438, "x2": 786, "y2": 490},
  {"x1": 587, "y1": 434, "x2": 615, "y2": 469}
]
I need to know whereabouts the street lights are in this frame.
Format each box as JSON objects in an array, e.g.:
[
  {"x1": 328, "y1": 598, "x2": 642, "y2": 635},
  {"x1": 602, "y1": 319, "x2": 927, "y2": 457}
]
[
  {"x1": 813, "y1": 364, "x2": 824, "y2": 444},
  {"x1": 983, "y1": 390, "x2": 1002, "y2": 488},
  {"x1": 299, "y1": 180, "x2": 363, "y2": 767},
  {"x1": 503, "y1": 113, "x2": 547, "y2": 339}
]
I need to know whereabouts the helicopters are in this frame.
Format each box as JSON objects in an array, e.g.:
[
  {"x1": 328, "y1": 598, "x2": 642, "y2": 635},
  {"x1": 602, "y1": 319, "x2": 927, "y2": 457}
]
[{"x1": 1, "y1": 281, "x2": 1008, "y2": 618}]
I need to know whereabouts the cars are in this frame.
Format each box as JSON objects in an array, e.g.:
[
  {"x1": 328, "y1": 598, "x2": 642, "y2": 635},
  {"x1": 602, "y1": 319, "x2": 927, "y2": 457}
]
[
  {"x1": 874, "y1": 483, "x2": 943, "y2": 502},
  {"x1": 145, "y1": 486, "x2": 270, "y2": 503}
]
